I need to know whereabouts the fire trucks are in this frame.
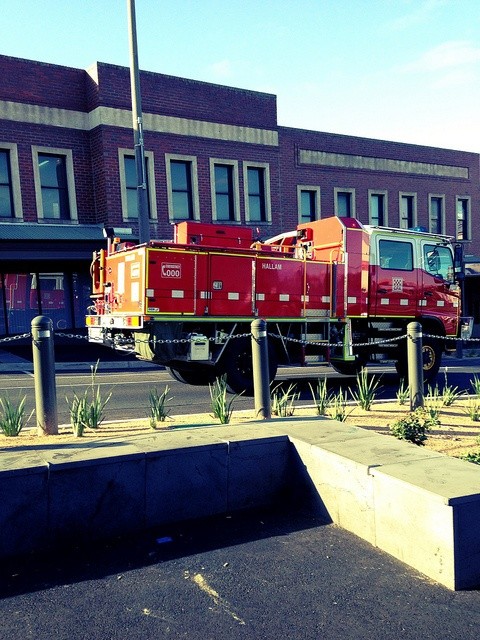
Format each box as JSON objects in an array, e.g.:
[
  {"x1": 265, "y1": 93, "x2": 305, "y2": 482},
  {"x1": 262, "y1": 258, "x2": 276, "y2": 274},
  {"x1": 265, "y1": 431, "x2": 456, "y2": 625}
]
[{"x1": 86, "y1": 217, "x2": 463, "y2": 395}]
